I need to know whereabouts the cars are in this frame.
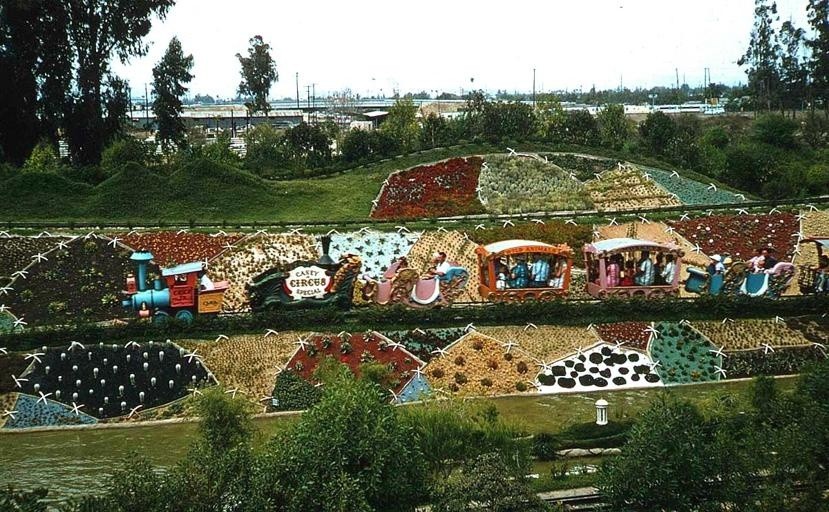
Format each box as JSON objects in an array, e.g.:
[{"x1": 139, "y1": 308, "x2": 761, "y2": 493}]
[{"x1": 704, "y1": 107, "x2": 725, "y2": 115}]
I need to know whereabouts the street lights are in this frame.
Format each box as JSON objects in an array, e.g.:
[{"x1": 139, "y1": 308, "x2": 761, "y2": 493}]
[{"x1": 648, "y1": 94, "x2": 658, "y2": 114}]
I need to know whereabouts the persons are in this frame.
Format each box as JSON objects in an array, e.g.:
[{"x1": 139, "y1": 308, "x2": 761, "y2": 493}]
[
  {"x1": 594, "y1": 251, "x2": 676, "y2": 287},
  {"x1": 704, "y1": 249, "x2": 778, "y2": 274},
  {"x1": 428, "y1": 253, "x2": 450, "y2": 276},
  {"x1": 494, "y1": 253, "x2": 567, "y2": 290}
]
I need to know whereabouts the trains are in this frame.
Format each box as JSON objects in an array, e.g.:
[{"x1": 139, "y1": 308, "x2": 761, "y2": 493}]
[{"x1": 121, "y1": 237, "x2": 829, "y2": 328}]
